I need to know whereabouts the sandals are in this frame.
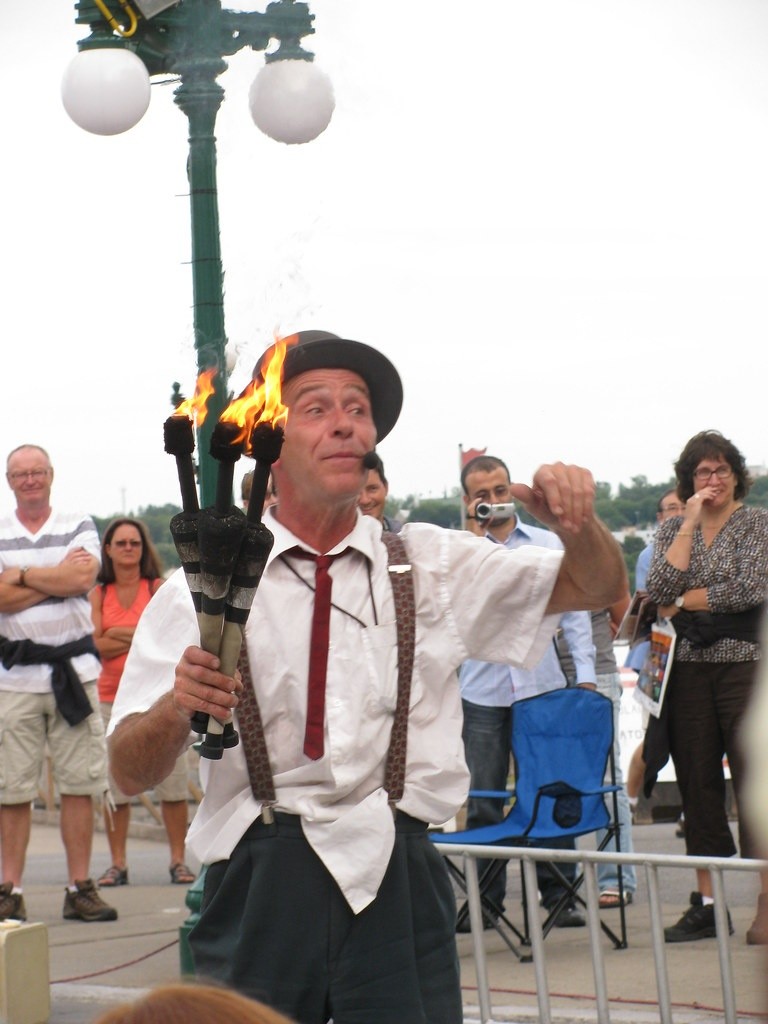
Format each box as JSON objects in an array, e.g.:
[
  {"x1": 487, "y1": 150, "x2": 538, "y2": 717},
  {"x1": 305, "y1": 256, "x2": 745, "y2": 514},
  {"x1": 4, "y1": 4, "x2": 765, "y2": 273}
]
[
  {"x1": 98, "y1": 865, "x2": 128, "y2": 887},
  {"x1": 598, "y1": 888, "x2": 632, "y2": 907},
  {"x1": 170, "y1": 863, "x2": 198, "y2": 883}
]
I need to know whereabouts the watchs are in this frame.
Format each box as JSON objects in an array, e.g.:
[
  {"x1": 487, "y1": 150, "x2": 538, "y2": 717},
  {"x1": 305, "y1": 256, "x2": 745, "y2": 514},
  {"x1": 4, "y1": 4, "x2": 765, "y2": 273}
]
[
  {"x1": 675, "y1": 589, "x2": 694, "y2": 613},
  {"x1": 19, "y1": 565, "x2": 31, "y2": 587}
]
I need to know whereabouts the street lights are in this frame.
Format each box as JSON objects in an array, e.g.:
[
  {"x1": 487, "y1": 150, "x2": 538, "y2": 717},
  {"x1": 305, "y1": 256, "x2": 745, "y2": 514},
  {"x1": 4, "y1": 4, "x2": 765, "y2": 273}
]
[{"x1": 63, "y1": 0, "x2": 339, "y2": 982}]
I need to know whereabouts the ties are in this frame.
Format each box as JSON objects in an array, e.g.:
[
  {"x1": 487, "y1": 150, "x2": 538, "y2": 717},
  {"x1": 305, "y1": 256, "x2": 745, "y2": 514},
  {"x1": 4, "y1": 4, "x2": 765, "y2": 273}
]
[{"x1": 287, "y1": 550, "x2": 352, "y2": 759}]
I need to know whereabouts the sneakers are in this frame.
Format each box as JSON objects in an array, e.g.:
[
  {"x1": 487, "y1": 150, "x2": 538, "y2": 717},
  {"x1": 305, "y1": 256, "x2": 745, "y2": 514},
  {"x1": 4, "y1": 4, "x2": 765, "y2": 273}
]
[
  {"x1": 64, "y1": 878, "x2": 116, "y2": 919},
  {"x1": 663, "y1": 891, "x2": 735, "y2": 941},
  {"x1": 0, "y1": 882, "x2": 25, "y2": 921}
]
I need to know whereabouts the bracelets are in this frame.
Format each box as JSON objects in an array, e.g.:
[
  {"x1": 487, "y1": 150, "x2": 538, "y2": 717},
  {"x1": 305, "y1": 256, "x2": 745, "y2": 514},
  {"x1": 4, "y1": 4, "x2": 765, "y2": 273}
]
[{"x1": 676, "y1": 532, "x2": 694, "y2": 538}]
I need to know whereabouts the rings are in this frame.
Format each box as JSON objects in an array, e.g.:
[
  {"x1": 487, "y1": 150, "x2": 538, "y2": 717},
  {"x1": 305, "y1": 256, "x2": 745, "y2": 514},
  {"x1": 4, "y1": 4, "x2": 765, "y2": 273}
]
[{"x1": 693, "y1": 493, "x2": 700, "y2": 499}]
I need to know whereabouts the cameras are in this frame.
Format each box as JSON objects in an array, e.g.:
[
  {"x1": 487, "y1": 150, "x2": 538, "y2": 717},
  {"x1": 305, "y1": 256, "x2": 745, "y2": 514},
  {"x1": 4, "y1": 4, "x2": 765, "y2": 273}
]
[{"x1": 475, "y1": 503, "x2": 516, "y2": 520}]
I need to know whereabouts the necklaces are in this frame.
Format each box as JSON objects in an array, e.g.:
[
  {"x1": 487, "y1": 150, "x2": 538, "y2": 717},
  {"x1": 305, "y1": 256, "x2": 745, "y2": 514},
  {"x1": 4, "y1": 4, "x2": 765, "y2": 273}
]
[{"x1": 698, "y1": 500, "x2": 739, "y2": 529}]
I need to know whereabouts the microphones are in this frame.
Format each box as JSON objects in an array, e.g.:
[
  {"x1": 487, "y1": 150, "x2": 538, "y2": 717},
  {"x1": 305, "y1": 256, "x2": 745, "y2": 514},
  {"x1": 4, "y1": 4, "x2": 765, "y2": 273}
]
[{"x1": 363, "y1": 448, "x2": 378, "y2": 469}]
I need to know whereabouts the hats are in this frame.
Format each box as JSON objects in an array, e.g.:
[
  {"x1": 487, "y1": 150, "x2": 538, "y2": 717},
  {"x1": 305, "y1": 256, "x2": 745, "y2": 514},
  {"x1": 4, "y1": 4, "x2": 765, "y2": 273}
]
[{"x1": 238, "y1": 331, "x2": 404, "y2": 444}]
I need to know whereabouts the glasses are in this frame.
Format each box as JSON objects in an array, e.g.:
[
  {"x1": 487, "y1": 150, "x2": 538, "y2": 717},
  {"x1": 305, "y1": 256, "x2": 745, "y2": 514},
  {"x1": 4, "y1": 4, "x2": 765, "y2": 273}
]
[
  {"x1": 10, "y1": 468, "x2": 52, "y2": 482},
  {"x1": 111, "y1": 540, "x2": 141, "y2": 547},
  {"x1": 692, "y1": 465, "x2": 733, "y2": 479}
]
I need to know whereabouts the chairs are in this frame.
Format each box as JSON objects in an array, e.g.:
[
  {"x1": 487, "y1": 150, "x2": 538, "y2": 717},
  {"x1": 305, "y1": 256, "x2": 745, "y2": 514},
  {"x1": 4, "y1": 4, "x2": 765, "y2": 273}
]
[{"x1": 427, "y1": 688, "x2": 629, "y2": 961}]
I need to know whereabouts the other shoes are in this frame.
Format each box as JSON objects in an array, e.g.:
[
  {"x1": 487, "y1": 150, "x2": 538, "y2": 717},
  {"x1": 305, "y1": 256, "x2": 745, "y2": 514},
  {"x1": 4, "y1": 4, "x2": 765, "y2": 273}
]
[
  {"x1": 457, "y1": 905, "x2": 498, "y2": 931},
  {"x1": 549, "y1": 901, "x2": 587, "y2": 927}
]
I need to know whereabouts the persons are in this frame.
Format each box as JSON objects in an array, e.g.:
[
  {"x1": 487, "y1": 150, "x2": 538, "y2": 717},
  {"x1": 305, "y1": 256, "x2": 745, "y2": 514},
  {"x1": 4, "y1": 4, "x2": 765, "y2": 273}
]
[
  {"x1": 643, "y1": 428, "x2": 768, "y2": 945},
  {"x1": 105, "y1": 330, "x2": 631, "y2": 1024},
  {"x1": 0, "y1": 443, "x2": 117, "y2": 921},
  {"x1": 455, "y1": 456, "x2": 597, "y2": 933},
  {"x1": 85, "y1": 519, "x2": 197, "y2": 886},
  {"x1": 0, "y1": 329, "x2": 768, "y2": 1024}
]
